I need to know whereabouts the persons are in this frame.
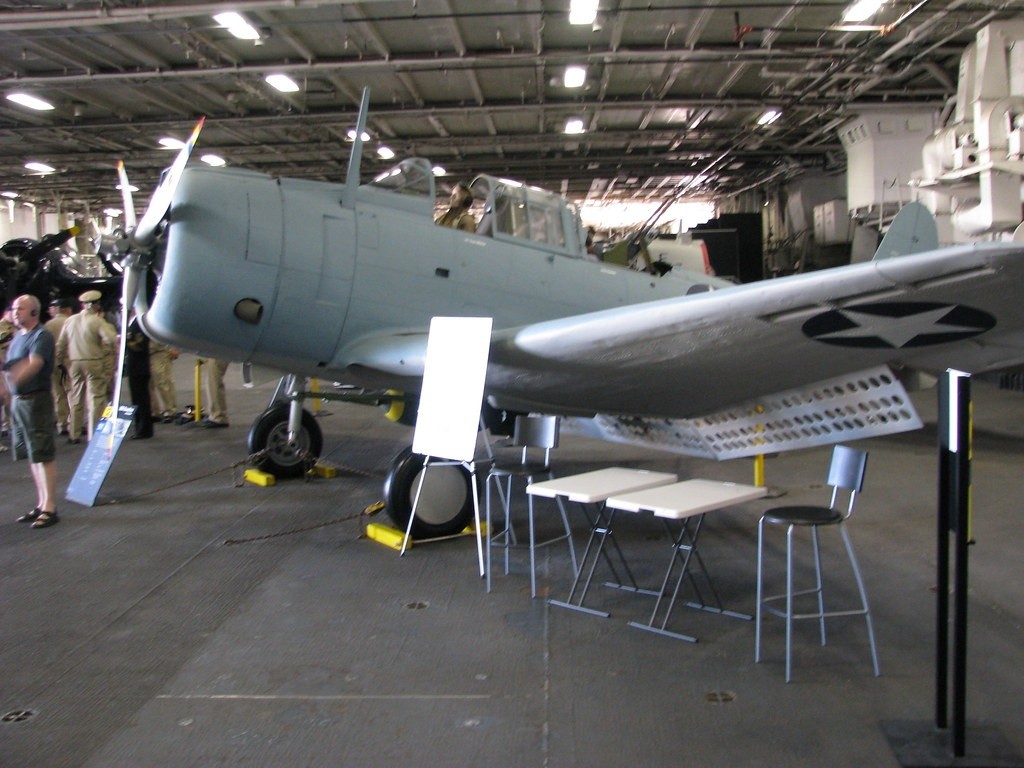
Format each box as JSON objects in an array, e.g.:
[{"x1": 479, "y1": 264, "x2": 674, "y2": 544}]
[
  {"x1": 200, "y1": 358, "x2": 232, "y2": 428},
  {"x1": 126, "y1": 318, "x2": 153, "y2": 439},
  {"x1": 4, "y1": 294, "x2": 57, "y2": 528},
  {"x1": 0, "y1": 290, "x2": 118, "y2": 452},
  {"x1": 151, "y1": 340, "x2": 179, "y2": 422},
  {"x1": 434, "y1": 182, "x2": 476, "y2": 233}
]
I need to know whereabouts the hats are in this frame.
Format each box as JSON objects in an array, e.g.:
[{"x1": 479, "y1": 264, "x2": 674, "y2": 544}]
[{"x1": 78, "y1": 289, "x2": 102, "y2": 303}]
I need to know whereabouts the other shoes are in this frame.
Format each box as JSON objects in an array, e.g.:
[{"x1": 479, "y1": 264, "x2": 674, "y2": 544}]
[
  {"x1": 131, "y1": 432, "x2": 150, "y2": 439},
  {"x1": 164, "y1": 414, "x2": 178, "y2": 422},
  {"x1": 153, "y1": 416, "x2": 163, "y2": 422},
  {"x1": 69, "y1": 437, "x2": 81, "y2": 444},
  {"x1": 58, "y1": 430, "x2": 67, "y2": 436},
  {"x1": 204, "y1": 420, "x2": 230, "y2": 428},
  {"x1": 2, "y1": 430, "x2": 8, "y2": 437}
]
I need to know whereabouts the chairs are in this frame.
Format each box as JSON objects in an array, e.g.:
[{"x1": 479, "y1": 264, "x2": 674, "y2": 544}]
[
  {"x1": 484, "y1": 414, "x2": 579, "y2": 595},
  {"x1": 476, "y1": 199, "x2": 509, "y2": 235},
  {"x1": 754, "y1": 444, "x2": 880, "y2": 683}
]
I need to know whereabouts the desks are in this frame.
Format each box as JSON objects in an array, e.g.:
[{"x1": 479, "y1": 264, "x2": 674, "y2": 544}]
[
  {"x1": 526, "y1": 466, "x2": 679, "y2": 618},
  {"x1": 606, "y1": 478, "x2": 767, "y2": 644}
]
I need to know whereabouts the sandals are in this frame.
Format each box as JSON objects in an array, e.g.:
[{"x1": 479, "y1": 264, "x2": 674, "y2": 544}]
[
  {"x1": 32, "y1": 509, "x2": 57, "y2": 528},
  {"x1": 17, "y1": 507, "x2": 42, "y2": 522}
]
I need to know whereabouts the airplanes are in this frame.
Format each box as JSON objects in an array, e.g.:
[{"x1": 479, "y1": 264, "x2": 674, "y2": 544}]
[
  {"x1": 0, "y1": 225, "x2": 156, "y2": 323},
  {"x1": 96, "y1": 85, "x2": 1024, "y2": 541}
]
